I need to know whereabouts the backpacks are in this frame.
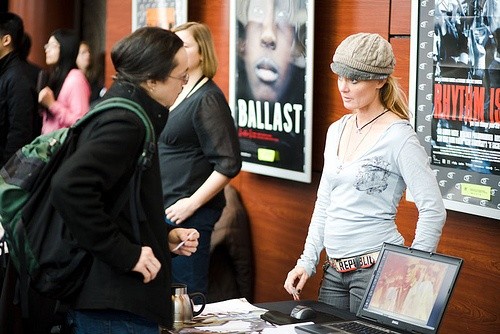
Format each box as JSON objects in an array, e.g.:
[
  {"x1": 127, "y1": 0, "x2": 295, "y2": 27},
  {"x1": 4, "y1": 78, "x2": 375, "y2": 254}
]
[{"x1": 0, "y1": 97, "x2": 156, "y2": 316}]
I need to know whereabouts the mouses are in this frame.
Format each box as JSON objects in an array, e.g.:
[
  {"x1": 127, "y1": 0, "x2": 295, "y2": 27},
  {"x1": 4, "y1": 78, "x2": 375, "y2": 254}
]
[{"x1": 289, "y1": 305, "x2": 315, "y2": 320}]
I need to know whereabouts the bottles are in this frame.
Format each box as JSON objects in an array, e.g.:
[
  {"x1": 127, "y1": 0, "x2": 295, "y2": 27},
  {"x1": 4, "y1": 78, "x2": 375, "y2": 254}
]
[{"x1": 35, "y1": 137, "x2": 58, "y2": 163}]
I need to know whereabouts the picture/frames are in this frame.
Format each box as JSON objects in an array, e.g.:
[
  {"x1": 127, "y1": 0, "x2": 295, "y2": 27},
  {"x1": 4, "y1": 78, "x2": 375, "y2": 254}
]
[
  {"x1": 227, "y1": 0, "x2": 315, "y2": 183},
  {"x1": 132, "y1": 0, "x2": 188, "y2": 33},
  {"x1": 405, "y1": 0, "x2": 500, "y2": 220}
]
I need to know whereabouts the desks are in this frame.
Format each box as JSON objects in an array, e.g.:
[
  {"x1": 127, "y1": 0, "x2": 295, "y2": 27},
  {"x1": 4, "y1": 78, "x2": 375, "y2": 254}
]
[{"x1": 177, "y1": 300, "x2": 431, "y2": 334}]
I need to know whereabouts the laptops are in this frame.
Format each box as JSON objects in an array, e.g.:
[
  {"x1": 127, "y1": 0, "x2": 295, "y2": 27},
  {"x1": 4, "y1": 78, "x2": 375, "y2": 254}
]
[{"x1": 296, "y1": 241, "x2": 463, "y2": 334}]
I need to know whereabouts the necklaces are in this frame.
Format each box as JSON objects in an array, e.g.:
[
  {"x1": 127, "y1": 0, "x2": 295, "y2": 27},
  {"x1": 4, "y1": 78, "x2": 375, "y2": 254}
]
[{"x1": 355, "y1": 109, "x2": 390, "y2": 134}]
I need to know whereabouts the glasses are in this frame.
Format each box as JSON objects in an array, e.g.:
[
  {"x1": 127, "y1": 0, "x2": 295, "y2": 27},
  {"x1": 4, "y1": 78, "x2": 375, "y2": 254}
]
[
  {"x1": 170, "y1": 73, "x2": 189, "y2": 85},
  {"x1": 44, "y1": 44, "x2": 60, "y2": 49}
]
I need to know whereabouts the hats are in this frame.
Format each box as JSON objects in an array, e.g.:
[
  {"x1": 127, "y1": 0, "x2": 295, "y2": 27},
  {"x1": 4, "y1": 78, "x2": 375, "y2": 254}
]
[{"x1": 330, "y1": 33, "x2": 396, "y2": 80}]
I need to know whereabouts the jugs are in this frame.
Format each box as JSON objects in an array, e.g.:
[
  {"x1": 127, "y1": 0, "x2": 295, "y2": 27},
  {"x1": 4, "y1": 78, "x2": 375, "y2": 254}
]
[{"x1": 170, "y1": 284, "x2": 207, "y2": 329}]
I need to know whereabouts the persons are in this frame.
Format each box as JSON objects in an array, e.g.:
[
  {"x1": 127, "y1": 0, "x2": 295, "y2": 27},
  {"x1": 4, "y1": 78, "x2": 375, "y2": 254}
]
[
  {"x1": 76, "y1": 41, "x2": 100, "y2": 105},
  {"x1": 384, "y1": 256, "x2": 433, "y2": 320},
  {"x1": 157, "y1": 22, "x2": 243, "y2": 305},
  {"x1": 0, "y1": 12, "x2": 42, "y2": 169},
  {"x1": 39, "y1": 30, "x2": 92, "y2": 134},
  {"x1": 283, "y1": 32, "x2": 447, "y2": 312},
  {"x1": 51, "y1": 27, "x2": 200, "y2": 334},
  {"x1": 237, "y1": 0, "x2": 308, "y2": 105}
]
